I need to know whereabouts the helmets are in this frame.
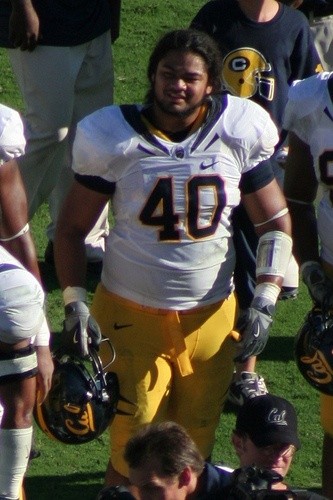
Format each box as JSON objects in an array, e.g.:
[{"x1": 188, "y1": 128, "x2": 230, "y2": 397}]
[
  {"x1": 34, "y1": 360, "x2": 120, "y2": 445},
  {"x1": 294, "y1": 305, "x2": 332, "y2": 397}
]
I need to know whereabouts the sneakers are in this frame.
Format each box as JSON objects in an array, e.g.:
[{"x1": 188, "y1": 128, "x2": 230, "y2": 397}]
[{"x1": 224, "y1": 372, "x2": 271, "y2": 414}]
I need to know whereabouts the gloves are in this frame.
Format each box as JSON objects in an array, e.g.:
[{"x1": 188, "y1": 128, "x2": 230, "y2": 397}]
[
  {"x1": 232, "y1": 296, "x2": 275, "y2": 364},
  {"x1": 62, "y1": 301, "x2": 102, "y2": 360}
]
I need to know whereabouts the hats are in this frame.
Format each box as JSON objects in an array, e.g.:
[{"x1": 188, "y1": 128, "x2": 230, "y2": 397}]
[{"x1": 234, "y1": 393, "x2": 301, "y2": 452}]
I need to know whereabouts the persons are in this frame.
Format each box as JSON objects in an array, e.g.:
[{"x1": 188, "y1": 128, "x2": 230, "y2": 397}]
[
  {"x1": 0, "y1": 103, "x2": 53, "y2": 405},
  {"x1": 206, "y1": 392, "x2": 299, "y2": 481},
  {"x1": 0, "y1": 1, "x2": 120, "y2": 281},
  {"x1": 122, "y1": 422, "x2": 330, "y2": 500},
  {"x1": 183, "y1": 0, "x2": 316, "y2": 298},
  {"x1": 52, "y1": 29, "x2": 293, "y2": 488},
  {"x1": 0, "y1": 245, "x2": 45, "y2": 499},
  {"x1": 227, "y1": 205, "x2": 268, "y2": 405},
  {"x1": 281, "y1": 74, "x2": 331, "y2": 499}
]
